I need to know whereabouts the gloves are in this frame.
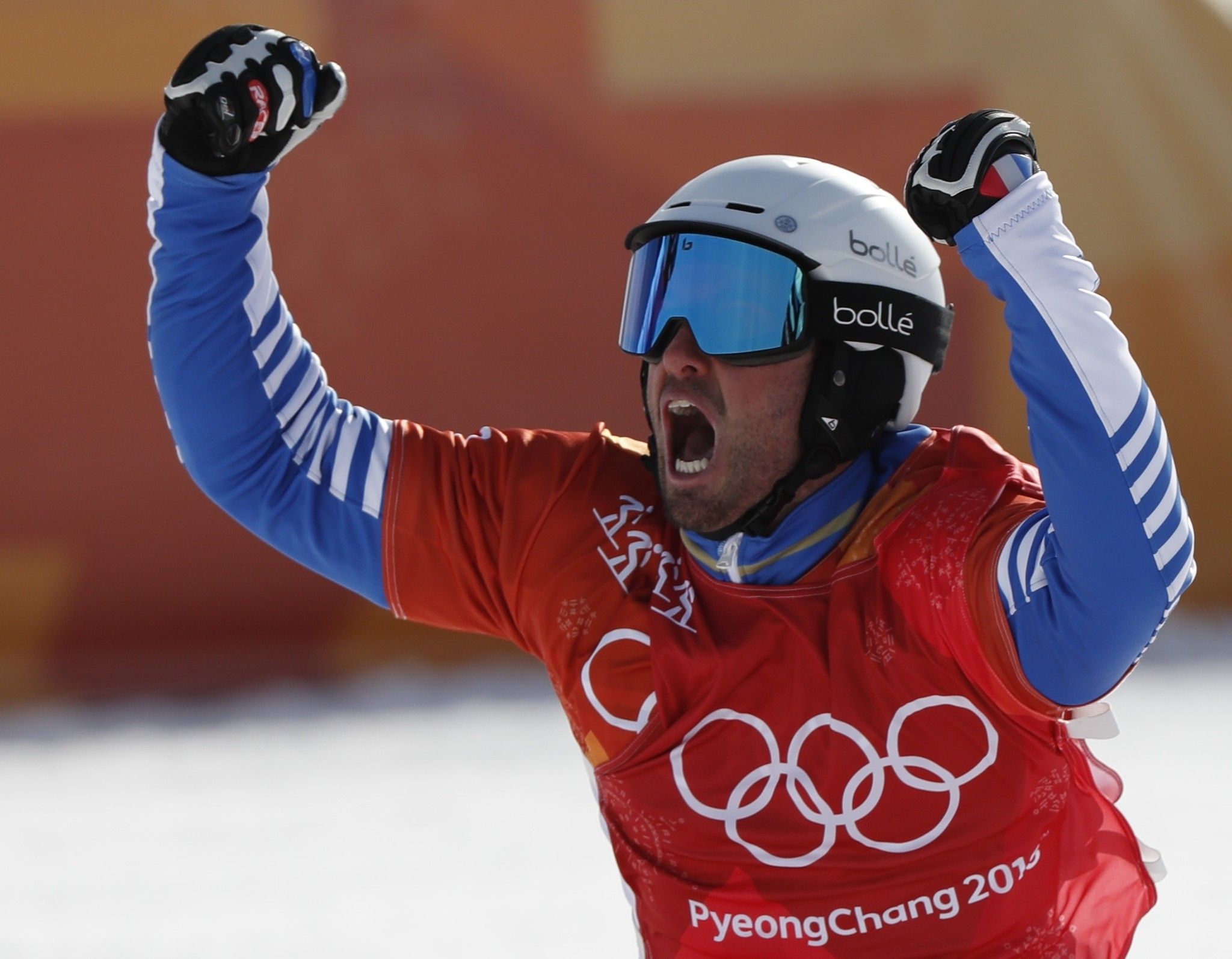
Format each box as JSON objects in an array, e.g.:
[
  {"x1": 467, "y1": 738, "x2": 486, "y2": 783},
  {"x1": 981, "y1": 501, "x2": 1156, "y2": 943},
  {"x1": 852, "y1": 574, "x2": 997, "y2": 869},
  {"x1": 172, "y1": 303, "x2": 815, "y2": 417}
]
[
  {"x1": 154, "y1": 25, "x2": 349, "y2": 178},
  {"x1": 903, "y1": 107, "x2": 1041, "y2": 246}
]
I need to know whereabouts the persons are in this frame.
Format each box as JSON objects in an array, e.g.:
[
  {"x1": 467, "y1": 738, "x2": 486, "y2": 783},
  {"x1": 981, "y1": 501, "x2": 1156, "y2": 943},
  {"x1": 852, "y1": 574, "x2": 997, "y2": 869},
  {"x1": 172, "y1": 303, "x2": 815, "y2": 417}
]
[{"x1": 137, "y1": 18, "x2": 1202, "y2": 959}]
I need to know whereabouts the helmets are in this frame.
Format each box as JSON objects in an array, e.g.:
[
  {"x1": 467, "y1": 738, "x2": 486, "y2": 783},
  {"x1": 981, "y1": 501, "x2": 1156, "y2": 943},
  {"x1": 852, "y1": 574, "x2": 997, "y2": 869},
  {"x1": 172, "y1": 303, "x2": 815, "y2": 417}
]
[{"x1": 626, "y1": 154, "x2": 953, "y2": 453}]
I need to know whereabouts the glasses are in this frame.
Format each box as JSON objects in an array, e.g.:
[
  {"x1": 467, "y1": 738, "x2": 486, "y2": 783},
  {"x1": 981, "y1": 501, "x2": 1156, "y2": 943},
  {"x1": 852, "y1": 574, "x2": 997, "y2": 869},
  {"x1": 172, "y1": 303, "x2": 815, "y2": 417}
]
[{"x1": 618, "y1": 228, "x2": 813, "y2": 367}]
